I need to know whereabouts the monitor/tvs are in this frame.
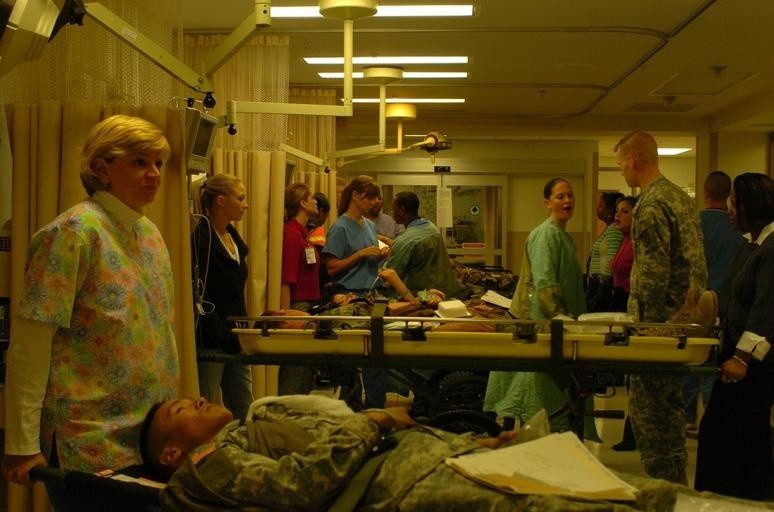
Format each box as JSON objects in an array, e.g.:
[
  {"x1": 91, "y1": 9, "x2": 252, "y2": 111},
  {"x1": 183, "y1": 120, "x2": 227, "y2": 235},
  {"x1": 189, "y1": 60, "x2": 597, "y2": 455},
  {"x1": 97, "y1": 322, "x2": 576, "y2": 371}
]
[{"x1": 184, "y1": 106, "x2": 220, "y2": 174}]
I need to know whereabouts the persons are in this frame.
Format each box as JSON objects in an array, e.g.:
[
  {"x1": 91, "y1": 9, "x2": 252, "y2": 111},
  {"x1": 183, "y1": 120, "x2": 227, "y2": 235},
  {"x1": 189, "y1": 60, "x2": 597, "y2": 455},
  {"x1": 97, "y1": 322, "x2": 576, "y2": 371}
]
[
  {"x1": 191, "y1": 173, "x2": 254, "y2": 426},
  {"x1": 1, "y1": 114, "x2": 182, "y2": 512},
  {"x1": 511, "y1": 177, "x2": 587, "y2": 335},
  {"x1": 137, "y1": 396, "x2": 773, "y2": 512},
  {"x1": 278, "y1": 170, "x2": 464, "y2": 415},
  {"x1": 684, "y1": 170, "x2": 774, "y2": 500},
  {"x1": 585, "y1": 189, "x2": 637, "y2": 313},
  {"x1": 614, "y1": 130, "x2": 708, "y2": 486}
]
